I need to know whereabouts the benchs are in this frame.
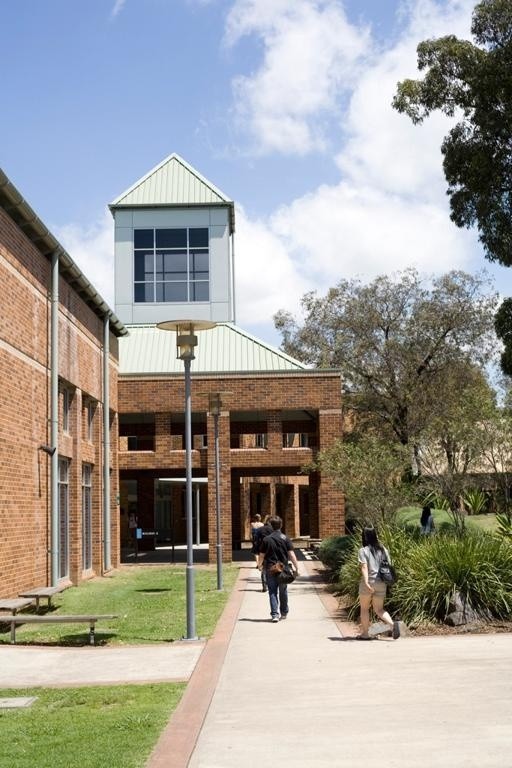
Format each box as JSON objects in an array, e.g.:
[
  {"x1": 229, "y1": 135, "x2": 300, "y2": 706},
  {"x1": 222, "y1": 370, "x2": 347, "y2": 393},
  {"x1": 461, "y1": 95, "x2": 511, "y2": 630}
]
[
  {"x1": 18, "y1": 586, "x2": 60, "y2": 613},
  {"x1": 0, "y1": 615, "x2": 118, "y2": 645},
  {"x1": 0, "y1": 598, "x2": 34, "y2": 616}
]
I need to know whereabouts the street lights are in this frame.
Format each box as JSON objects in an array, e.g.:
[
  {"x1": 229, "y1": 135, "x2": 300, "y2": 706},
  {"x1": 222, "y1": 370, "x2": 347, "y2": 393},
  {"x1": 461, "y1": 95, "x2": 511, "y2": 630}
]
[{"x1": 156, "y1": 318, "x2": 233, "y2": 640}]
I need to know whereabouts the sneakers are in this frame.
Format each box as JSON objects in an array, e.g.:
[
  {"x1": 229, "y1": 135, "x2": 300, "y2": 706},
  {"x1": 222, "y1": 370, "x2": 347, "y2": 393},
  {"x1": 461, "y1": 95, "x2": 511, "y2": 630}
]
[
  {"x1": 272, "y1": 614, "x2": 286, "y2": 622},
  {"x1": 354, "y1": 634, "x2": 371, "y2": 640},
  {"x1": 391, "y1": 622, "x2": 401, "y2": 640}
]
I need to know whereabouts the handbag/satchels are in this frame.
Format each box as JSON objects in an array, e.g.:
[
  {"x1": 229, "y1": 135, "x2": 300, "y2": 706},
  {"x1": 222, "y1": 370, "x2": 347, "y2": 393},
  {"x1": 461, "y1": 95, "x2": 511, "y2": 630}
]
[
  {"x1": 273, "y1": 562, "x2": 297, "y2": 584},
  {"x1": 377, "y1": 547, "x2": 398, "y2": 584}
]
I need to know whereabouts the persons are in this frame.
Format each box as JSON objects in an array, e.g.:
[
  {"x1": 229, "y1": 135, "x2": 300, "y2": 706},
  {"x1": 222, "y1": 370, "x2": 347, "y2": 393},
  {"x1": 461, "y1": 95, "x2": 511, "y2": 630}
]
[
  {"x1": 356, "y1": 523, "x2": 401, "y2": 640},
  {"x1": 256, "y1": 513, "x2": 301, "y2": 622},
  {"x1": 127, "y1": 509, "x2": 139, "y2": 545},
  {"x1": 249, "y1": 513, "x2": 273, "y2": 592},
  {"x1": 419, "y1": 504, "x2": 436, "y2": 533},
  {"x1": 249, "y1": 512, "x2": 264, "y2": 569}
]
